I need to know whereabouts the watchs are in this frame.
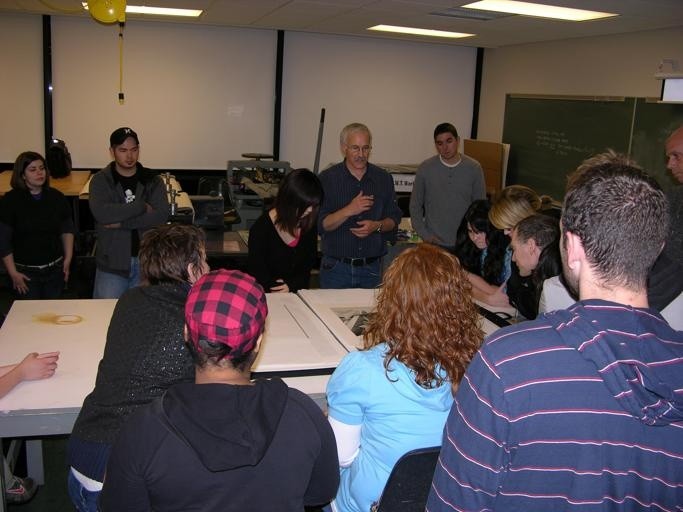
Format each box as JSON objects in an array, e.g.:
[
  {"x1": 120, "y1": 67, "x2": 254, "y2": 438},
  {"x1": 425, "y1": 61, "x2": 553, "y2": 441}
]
[{"x1": 376, "y1": 221, "x2": 384, "y2": 232}]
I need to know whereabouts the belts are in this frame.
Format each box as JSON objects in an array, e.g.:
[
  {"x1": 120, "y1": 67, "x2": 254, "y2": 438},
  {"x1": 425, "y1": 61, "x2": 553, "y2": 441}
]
[{"x1": 324, "y1": 252, "x2": 381, "y2": 267}]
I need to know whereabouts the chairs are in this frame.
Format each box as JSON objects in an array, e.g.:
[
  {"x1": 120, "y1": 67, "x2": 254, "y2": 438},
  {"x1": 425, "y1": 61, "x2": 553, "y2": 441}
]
[{"x1": 377, "y1": 445, "x2": 440, "y2": 512}]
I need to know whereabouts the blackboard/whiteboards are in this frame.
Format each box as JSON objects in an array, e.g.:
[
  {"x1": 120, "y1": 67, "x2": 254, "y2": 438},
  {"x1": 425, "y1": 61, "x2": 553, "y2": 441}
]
[{"x1": 501, "y1": 93, "x2": 683, "y2": 232}]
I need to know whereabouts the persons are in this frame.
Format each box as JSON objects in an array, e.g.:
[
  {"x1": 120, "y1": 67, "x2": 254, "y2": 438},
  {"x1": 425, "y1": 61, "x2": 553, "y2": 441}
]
[
  {"x1": 1, "y1": 152, "x2": 76, "y2": 325},
  {"x1": 425, "y1": 147, "x2": 683, "y2": 512},
  {"x1": 89, "y1": 127, "x2": 170, "y2": 299},
  {"x1": 455, "y1": 123, "x2": 683, "y2": 331},
  {"x1": 325, "y1": 242, "x2": 487, "y2": 512},
  {"x1": 67, "y1": 224, "x2": 341, "y2": 511},
  {"x1": 409, "y1": 123, "x2": 486, "y2": 254},
  {"x1": 0, "y1": 352, "x2": 60, "y2": 504},
  {"x1": 248, "y1": 168, "x2": 325, "y2": 294},
  {"x1": 316, "y1": 123, "x2": 402, "y2": 288}
]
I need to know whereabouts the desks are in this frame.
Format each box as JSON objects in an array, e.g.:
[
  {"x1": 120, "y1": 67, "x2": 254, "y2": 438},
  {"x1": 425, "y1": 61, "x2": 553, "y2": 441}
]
[
  {"x1": 0, "y1": 292, "x2": 349, "y2": 486},
  {"x1": 299, "y1": 286, "x2": 514, "y2": 353},
  {"x1": 0, "y1": 170, "x2": 90, "y2": 253}
]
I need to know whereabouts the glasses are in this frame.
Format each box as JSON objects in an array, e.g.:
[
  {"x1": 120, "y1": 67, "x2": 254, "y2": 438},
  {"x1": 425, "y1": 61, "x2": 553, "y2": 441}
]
[{"x1": 350, "y1": 145, "x2": 373, "y2": 152}]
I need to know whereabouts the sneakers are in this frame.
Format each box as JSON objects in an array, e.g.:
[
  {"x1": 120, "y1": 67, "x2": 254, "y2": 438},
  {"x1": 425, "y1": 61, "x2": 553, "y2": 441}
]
[{"x1": 6, "y1": 477, "x2": 37, "y2": 503}]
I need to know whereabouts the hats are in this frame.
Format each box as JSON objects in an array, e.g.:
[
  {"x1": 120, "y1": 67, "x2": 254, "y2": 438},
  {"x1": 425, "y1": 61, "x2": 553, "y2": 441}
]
[
  {"x1": 109, "y1": 127, "x2": 139, "y2": 146},
  {"x1": 185, "y1": 268, "x2": 268, "y2": 359}
]
